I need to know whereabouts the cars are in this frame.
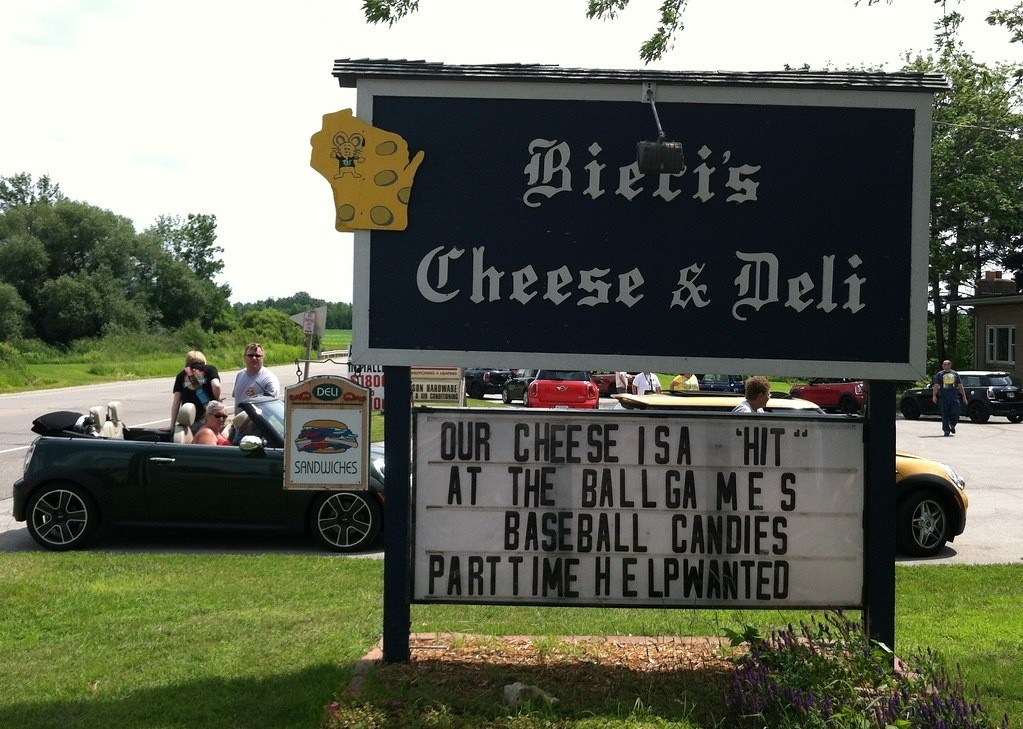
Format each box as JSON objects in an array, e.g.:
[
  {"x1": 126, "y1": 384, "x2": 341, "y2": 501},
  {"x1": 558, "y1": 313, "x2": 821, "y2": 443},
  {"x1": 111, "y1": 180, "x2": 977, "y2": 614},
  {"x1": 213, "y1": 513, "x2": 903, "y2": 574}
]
[
  {"x1": 899, "y1": 371, "x2": 1023, "y2": 423},
  {"x1": 695, "y1": 374, "x2": 749, "y2": 397},
  {"x1": 791, "y1": 378, "x2": 863, "y2": 414},
  {"x1": 589, "y1": 369, "x2": 644, "y2": 395},
  {"x1": 502, "y1": 368, "x2": 540, "y2": 407},
  {"x1": 608, "y1": 389, "x2": 970, "y2": 557},
  {"x1": 528, "y1": 368, "x2": 600, "y2": 409},
  {"x1": 464, "y1": 368, "x2": 518, "y2": 400}
]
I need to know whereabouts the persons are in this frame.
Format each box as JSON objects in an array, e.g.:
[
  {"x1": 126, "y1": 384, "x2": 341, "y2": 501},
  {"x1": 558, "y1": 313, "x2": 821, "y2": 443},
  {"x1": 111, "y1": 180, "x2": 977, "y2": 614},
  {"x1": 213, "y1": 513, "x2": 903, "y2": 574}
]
[
  {"x1": 615, "y1": 370, "x2": 630, "y2": 394},
  {"x1": 232, "y1": 343, "x2": 280, "y2": 417},
  {"x1": 933, "y1": 359, "x2": 968, "y2": 439},
  {"x1": 730, "y1": 375, "x2": 772, "y2": 414},
  {"x1": 158, "y1": 349, "x2": 227, "y2": 432},
  {"x1": 632, "y1": 370, "x2": 662, "y2": 394},
  {"x1": 191, "y1": 401, "x2": 236, "y2": 447},
  {"x1": 670, "y1": 372, "x2": 698, "y2": 394}
]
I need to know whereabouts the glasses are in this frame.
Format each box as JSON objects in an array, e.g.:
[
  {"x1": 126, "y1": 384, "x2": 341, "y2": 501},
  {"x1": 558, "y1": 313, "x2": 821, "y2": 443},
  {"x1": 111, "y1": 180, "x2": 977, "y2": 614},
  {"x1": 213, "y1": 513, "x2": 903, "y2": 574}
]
[
  {"x1": 245, "y1": 354, "x2": 263, "y2": 357},
  {"x1": 210, "y1": 413, "x2": 227, "y2": 418}
]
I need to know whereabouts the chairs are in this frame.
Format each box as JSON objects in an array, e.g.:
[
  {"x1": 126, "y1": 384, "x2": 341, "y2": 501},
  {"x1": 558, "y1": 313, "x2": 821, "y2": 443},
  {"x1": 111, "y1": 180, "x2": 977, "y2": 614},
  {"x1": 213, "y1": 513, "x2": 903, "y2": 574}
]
[
  {"x1": 88, "y1": 402, "x2": 127, "y2": 445},
  {"x1": 174, "y1": 401, "x2": 199, "y2": 444}
]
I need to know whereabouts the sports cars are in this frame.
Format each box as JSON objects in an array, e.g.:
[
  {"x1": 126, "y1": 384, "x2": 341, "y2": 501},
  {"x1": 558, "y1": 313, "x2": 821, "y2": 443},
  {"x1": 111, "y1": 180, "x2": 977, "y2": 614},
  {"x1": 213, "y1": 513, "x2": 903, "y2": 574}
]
[{"x1": 11, "y1": 391, "x2": 418, "y2": 552}]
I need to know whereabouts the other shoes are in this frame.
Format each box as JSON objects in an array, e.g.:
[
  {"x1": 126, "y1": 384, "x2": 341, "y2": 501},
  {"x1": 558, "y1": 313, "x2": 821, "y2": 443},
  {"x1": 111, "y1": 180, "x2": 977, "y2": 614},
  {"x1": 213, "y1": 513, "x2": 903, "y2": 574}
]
[
  {"x1": 950, "y1": 425, "x2": 956, "y2": 434},
  {"x1": 944, "y1": 430, "x2": 951, "y2": 436}
]
